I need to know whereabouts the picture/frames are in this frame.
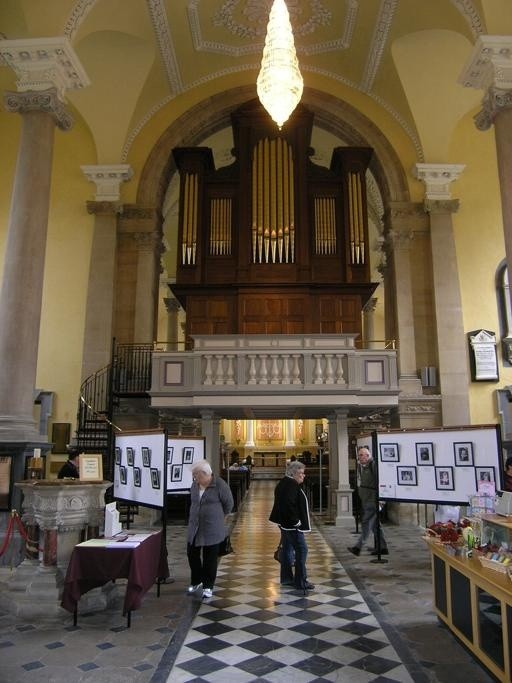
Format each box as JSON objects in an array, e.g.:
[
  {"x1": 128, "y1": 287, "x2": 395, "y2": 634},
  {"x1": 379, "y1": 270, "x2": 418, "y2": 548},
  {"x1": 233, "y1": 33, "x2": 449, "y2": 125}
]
[
  {"x1": 167, "y1": 446, "x2": 173, "y2": 464},
  {"x1": 378, "y1": 440, "x2": 497, "y2": 492},
  {"x1": 171, "y1": 463, "x2": 183, "y2": 482},
  {"x1": 78, "y1": 453, "x2": 103, "y2": 481},
  {"x1": 183, "y1": 446, "x2": 194, "y2": 463},
  {"x1": 114, "y1": 446, "x2": 160, "y2": 488}
]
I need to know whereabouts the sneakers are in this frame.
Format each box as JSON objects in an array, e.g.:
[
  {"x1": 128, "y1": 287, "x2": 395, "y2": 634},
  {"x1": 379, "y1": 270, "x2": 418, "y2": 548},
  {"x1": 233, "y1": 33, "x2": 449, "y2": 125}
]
[
  {"x1": 347, "y1": 546, "x2": 361, "y2": 556},
  {"x1": 202, "y1": 588, "x2": 213, "y2": 599},
  {"x1": 296, "y1": 582, "x2": 315, "y2": 590},
  {"x1": 371, "y1": 549, "x2": 389, "y2": 556},
  {"x1": 185, "y1": 582, "x2": 203, "y2": 593}
]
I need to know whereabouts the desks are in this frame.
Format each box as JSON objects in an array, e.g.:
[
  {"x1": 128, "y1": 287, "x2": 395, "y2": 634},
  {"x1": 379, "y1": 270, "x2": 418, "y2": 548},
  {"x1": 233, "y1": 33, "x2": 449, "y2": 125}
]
[{"x1": 60, "y1": 529, "x2": 169, "y2": 627}]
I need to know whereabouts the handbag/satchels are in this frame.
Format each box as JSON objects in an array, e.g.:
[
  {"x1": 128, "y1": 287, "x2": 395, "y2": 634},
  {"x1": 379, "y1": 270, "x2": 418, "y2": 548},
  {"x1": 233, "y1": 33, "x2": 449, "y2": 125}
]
[
  {"x1": 274, "y1": 546, "x2": 297, "y2": 567},
  {"x1": 217, "y1": 534, "x2": 234, "y2": 557}
]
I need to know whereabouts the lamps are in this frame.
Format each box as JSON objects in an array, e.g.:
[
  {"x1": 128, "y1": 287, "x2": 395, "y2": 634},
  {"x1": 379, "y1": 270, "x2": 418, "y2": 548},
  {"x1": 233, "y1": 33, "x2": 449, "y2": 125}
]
[{"x1": 254, "y1": 0, "x2": 305, "y2": 134}]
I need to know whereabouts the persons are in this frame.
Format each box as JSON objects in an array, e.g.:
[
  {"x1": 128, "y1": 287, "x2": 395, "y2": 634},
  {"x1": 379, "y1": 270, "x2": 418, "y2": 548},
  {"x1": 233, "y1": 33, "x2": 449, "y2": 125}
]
[
  {"x1": 184, "y1": 458, "x2": 236, "y2": 600},
  {"x1": 383, "y1": 447, "x2": 394, "y2": 457},
  {"x1": 401, "y1": 470, "x2": 412, "y2": 482},
  {"x1": 439, "y1": 470, "x2": 449, "y2": 484},
  {"x1": 115, "y1": 446, "x2": 193, "y2": 492},
  {"x1": 55, "y1": 448, "x2": 81, "y2": 481},
  {"x1": 344, "y1": 444, "x2": 389, "y2": 557},
  {"x1": 458, "y1": 446, "x2": 468, "y2": 460},
  {"x1": 501, "y1": 454, "x2": 512, "y2": 491},
  {"x1": 420, "y1": 447, "x2": 428, "y2": 460},
  {"x1": 268, "y1": 458, "x2": 316, "y2": 592},
  {"x1": 480, "y1": 471, "x2": 489, "y2": 480}
]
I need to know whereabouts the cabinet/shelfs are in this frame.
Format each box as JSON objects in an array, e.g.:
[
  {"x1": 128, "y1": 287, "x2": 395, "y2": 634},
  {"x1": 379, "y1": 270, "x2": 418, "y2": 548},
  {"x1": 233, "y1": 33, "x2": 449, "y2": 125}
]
[{"x1": 420, "y1": 511, "x2": 512, "y2": 681}]
